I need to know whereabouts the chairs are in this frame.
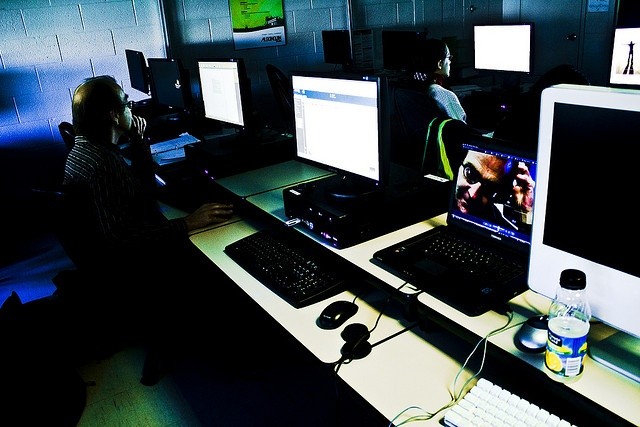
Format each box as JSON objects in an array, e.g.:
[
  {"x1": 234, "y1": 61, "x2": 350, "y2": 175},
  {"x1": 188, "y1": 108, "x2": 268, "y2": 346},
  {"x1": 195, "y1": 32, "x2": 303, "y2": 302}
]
[
  {"x1": 265, "y1": 64, "x2": 293, "y2": 129},
  {"x1": 32, "y1": 181, "x2": 105, "y2": 266},
  {"x1": 59, "y1": 122, "x2": 76, "y2": 151},
  {"x1": 386, "y1": 82, "x2": 456, "y2": 174}
]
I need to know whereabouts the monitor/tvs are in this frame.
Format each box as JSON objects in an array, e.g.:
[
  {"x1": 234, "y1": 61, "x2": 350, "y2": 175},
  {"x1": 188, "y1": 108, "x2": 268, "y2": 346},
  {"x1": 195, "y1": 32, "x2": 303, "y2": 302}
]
[
  {"x1": 526, "y1": 85, "x2": 637, "y2": 382},
  {"x1": 381, "y1": 31, "x2": 426, "y2": 75},
  {"x1": 289, "y1": 73, "x2": 385, "y2": 197},
  {"x1": 194, "y1": 57, "x2": 253, "y2": 149},
  {"x1": 470, "y1": 23, "x2": 533, "y2": 76},
  {"x1": 146, "y1": 58, "x2": 187, "y2": 109},
  {"x1": 126, "y1": 50, "x2": 150, "y2": 94},
  {"x1": 318, "y1": 30, "x2": 355, "y2": 67}
]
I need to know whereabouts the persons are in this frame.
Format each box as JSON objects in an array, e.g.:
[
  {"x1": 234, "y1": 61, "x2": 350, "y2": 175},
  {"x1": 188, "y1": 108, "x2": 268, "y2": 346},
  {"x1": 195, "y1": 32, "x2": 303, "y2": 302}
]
[
  {"x1": 61, "y1": 74, "x2": 235, "y2": 257},
  {"x1": 455, "y1": 150, "x2": 535, "y2": 235},
  {"x1": 399, "y1": 37, "x2": 509, "y2": 146}
]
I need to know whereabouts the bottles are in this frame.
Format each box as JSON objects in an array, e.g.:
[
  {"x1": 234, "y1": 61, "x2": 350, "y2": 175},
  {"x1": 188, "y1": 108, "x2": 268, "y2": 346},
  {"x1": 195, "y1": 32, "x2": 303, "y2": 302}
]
[{"x1": 544, "y1": 269, "x2": 592, "y2": 381}]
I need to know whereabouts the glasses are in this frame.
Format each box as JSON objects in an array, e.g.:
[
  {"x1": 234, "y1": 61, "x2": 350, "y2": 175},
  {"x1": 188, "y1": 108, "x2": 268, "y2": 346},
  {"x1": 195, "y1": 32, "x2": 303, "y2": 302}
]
[
  {"x1": 445, "y1": 55, "x2": 453, "y2": 60},
  {"x1": 462, "y1": 162, "x2": 503, "y2": 202},
  {"x1": 113, "y1": 100, "x2": 136, "y2": 109}
]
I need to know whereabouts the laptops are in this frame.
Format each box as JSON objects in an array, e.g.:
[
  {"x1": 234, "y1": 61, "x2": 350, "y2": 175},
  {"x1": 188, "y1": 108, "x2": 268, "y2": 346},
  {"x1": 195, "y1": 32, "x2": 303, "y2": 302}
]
[{"x1": 373, "y1": 143, "x2": 537, "y2": 318}]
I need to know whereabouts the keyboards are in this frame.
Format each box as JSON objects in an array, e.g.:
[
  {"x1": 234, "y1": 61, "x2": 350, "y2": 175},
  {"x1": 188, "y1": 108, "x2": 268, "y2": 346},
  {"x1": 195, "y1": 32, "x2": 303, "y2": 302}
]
[
  {"x1": 221, "y1": 232, "x2": 343, "y2": 310},
  {"x1": 440, "y1": 378, "x2": 587, "y2": 427}
]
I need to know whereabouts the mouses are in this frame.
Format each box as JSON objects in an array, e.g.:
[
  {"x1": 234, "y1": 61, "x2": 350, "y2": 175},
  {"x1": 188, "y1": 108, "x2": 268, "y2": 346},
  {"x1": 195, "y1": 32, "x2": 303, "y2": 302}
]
[{"x1": 313, "y1": 296, "x2": 356, "y2": 326}]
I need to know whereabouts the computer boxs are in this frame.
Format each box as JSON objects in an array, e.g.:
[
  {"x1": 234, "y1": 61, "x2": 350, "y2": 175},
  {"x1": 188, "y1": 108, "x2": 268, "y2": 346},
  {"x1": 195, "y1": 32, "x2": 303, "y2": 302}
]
[
  {"x1": 183, "y1": 133, "x2": 283, "y2": 181},
  {"x1": 283, "y1": 160, "x2": 449, "y2": 250}
]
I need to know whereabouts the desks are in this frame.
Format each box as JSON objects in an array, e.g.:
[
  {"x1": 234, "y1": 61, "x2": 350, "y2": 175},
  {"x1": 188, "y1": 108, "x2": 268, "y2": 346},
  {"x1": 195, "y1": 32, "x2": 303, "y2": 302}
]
[
  {"x1": 334, "y1": 289, "x2": 640, "y2": 427},
  {"x1": 132, "y1": 99, "x2": 334, "y2": 236},
  {"x1": 189, "y1": 173, "x2": 448, "y2": 364}
]
